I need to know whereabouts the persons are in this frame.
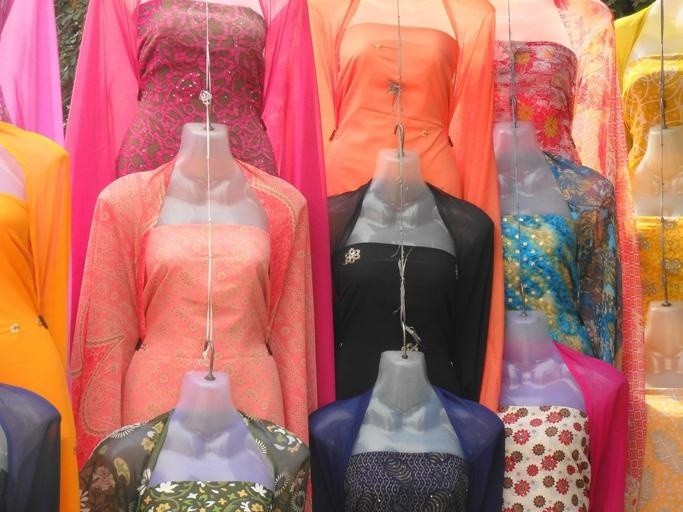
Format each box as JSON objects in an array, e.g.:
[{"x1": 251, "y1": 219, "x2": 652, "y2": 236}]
[
  {"x1": 308, "y1": 349, "x2": 506, "y2": 511},
  {"x1": 640, "y1": 300, "x2": 683, "y2": 512},
  {"x1": 496, "y1": 311, "x2": 628, "y2": 512},
  {"x1": 615, "y1": 0, "x2": 683, "y2": 180},
  {"x1": 493, "y1": 1, "x2": 645, "y2": 488},
  {"x1": 63, "y1": 2, "x2": 336, "y2": 409},
  {"x1": 1, "y1": 118, "x2": 80, "y2": 512},
  {"x1": 325, "y1": 152, "x2": 494, "y2": 406},
  {"x1": 70, "y1": 123, "x2": 317, "y2": 512},
  {"x1": 496, "y1": 124, "x2": 622, "y2": 376},
  {"x1": 634, "y1": 126, "x2": 683, "y2": 321},
  {"x1": 80, "y1": 369, "x2": 311, "y2": 512},
  {"x1": 309, "y1": 2, "x2": 504, "y2": 418}
]
